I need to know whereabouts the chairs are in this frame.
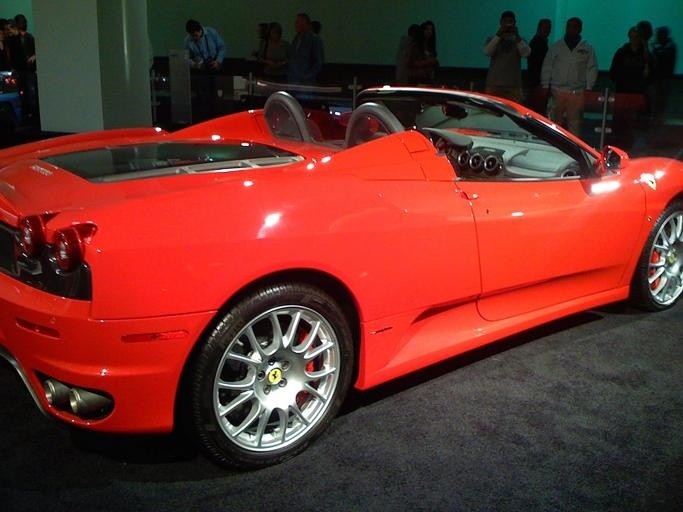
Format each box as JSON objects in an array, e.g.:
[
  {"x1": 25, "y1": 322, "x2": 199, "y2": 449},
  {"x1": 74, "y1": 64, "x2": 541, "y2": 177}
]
[{"x1": 261, "y1": 90, "x2": 405, "y2": 150}]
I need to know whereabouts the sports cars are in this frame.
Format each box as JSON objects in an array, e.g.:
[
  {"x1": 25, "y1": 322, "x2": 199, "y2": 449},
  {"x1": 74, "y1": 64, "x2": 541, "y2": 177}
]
[
  {"x1": 544, "y1": 85, "x2": 647, "y2": 133},
  {"x1": 0, "y1": 78, "x2": 683, "y2": 472}
]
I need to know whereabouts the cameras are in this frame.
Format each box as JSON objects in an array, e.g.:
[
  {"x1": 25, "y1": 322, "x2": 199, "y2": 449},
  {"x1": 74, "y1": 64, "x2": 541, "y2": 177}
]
[
  {"x1": 503, "y1": 24, "x2": 512, "y2": 29},
  {"x1": 207, "y1": 56, "x2": 216, "y2": 71}
]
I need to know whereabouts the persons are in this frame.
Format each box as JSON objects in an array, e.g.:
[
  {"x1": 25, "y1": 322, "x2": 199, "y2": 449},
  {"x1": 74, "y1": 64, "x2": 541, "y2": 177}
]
[
  {"x1": 608, "y1": 20, "x2": 677, "y2": 149},
  {"x1": 183, "y1": 19, "x2": 226, "y2": 79},
  {"x1": 528, "y1": 17, "x2": 599, "y2": 137},
  {"x1": 480, "y1": 11, "x2": 532, "y2": 97},
  {"x1": 0, "y1": 14, "x2": 37, "y2": 92},
  {"x1": 250, "y1": 13, "x2": 324, "y2": 84},
  {"x1": 396, "y1": 20, "x2": 439, "y2": 86}
]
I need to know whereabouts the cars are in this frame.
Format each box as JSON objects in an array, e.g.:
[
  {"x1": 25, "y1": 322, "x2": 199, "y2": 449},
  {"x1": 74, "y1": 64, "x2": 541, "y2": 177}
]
[{"x1": 0, "y1": 71, "x2": 36, "y2": 124}]
